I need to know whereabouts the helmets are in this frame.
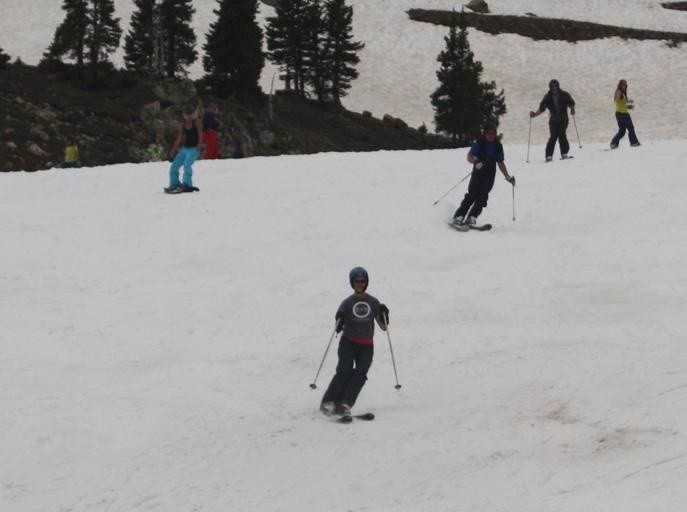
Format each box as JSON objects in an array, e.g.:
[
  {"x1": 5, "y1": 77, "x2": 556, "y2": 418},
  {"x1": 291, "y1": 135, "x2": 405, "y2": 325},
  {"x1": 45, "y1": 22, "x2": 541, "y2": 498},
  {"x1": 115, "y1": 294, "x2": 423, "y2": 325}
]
[{"x1": 350, "y1": 267, "x2": 368, "y2": 293}]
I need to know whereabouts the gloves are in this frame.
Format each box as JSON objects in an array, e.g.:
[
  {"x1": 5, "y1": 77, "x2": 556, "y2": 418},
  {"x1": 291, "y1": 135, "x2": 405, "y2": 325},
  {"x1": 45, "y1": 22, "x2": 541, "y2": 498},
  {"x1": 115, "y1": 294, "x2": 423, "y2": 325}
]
[
  {"x1": 336, "y1": 309, "x2": 344, "y2": 332},
  {"x1": 376, "y1": 304, "x2": 389, "y2": 325},
  {"x1": 506, "y1": 175, "x2": 515, "y2": 184},
  {"x1": 475, "y1": 162, "x2": 483, "y2": 170}
]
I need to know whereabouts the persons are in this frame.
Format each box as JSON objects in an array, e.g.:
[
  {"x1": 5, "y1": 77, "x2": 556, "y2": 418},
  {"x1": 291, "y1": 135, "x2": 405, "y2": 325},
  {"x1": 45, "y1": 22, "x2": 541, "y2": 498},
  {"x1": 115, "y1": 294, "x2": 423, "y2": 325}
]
[
  {"x1": 320, "y1": 266, "x2": 390, "y2": 418},
  {"x1": 63, "y1": 137, "x2": 80, "y2": 168},
  {"x1": 609, "y1": 79, "x2": 641, "y2": 150},
  {"x1": 163, "y1": 105, "x2": 204, "y2": 195},
  {"x1": 140, "y1": 106, "x2": 255, "y2": 163},
  {"x1": 451, "y1": 121, "x2": 516, "y2": 230},
  {"x1": 529, "y1": 79, "x2": 575, "y2": 163}
]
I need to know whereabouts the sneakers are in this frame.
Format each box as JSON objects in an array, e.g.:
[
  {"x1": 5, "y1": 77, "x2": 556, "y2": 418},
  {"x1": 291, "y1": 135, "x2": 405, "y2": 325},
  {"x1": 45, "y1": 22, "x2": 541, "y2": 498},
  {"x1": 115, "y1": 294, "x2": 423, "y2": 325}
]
[
  {"x1": 320, "y1": 400, "x2": 350, "y2": 416},
  {"x1": 465, "y1": 215, "x2": 476, "y2": 224},
  {"x1": 451, "y1": 216, "x2": 464, "y2": 226}
]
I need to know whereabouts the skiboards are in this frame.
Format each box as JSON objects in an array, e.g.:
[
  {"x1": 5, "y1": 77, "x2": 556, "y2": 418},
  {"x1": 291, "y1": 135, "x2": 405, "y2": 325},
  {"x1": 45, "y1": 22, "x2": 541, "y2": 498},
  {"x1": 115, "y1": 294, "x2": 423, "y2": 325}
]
[
  {"x1": 163, "y1": 184, "x2": 198, "y2": 194},
  {"x1": 313, "y1": 405, "x2": 375, "y2": 422},
  {"x1": 543, "y1": 155, "x2": 574, "y2": 162},
  {"x1": 445, "y1": 218, "x2": 492, "y2": 230}
]
